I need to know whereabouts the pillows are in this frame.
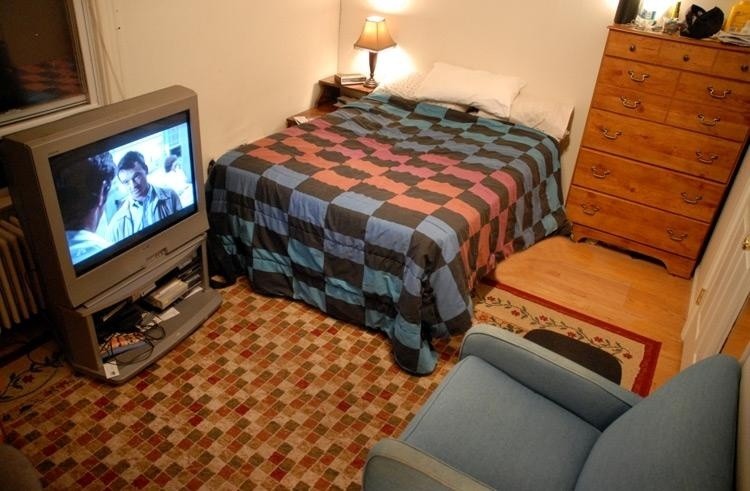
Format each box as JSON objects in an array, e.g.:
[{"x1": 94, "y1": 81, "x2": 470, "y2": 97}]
[{"x1": 387, "y1": 61, "x2": 577, "y2": 146}]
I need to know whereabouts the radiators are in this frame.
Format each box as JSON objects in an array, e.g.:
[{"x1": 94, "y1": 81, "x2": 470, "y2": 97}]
[{"x1": 0, "y1": 214, "x2": 47, "y2": 331}]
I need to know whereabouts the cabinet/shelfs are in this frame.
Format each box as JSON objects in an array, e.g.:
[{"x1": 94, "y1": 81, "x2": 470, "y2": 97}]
[
  {"x1": 564, "y1": 25, "x2": 750, "y2": 280},
  {"x1": 49, "y1": 236, "x2": 223, "y2": 386},
  {"x1": 286, "y1": 76, "x2": 379, "y2": 128}
]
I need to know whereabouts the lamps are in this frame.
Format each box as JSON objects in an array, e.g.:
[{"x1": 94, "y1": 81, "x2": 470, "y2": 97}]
[{"x1": 353, "y1": 16, "x2": 398, "y2": 89}]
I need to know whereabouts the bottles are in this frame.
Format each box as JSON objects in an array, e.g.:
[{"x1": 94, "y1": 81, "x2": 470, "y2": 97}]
[
  {"x1": 670, "y1": 1, "x2": 682, "y2": 22},
  {"x1": 634, "y1": 0, "x2": 661, "y2": 27},
  {"x1": 724, "y1": 1, "x2": 750, "y2": 34}
]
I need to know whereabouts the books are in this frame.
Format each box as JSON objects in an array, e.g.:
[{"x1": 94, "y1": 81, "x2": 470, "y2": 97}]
[
  {"x1": 710, "y1": 28, "x2": 749, "y2": 47},
  {"x1": 334, "y1": 73, "x2": 366, "y2": 108},
  {"x1": 99, "y1": 250, "x2": 204, "y2": 359}
]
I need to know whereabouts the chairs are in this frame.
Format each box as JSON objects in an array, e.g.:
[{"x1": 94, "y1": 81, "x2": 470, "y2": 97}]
[{"x1": 362, "y1": 324, "x2": 740, "y2": 491}]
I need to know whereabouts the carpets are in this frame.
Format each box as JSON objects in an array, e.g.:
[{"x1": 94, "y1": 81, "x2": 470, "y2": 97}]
[{"x1": 0, "y1": 278, "x2": 664, "y2": 490}]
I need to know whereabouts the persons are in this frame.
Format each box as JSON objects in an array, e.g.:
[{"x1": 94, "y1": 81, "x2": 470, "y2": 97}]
[
  {"x1": 157, "y1": 154, "x2": 186, "y2": 199},
  {"x1": 55, "y1": 152, "x2": 111, "y2": 263},
  {"x1": 105, "y1": 150, "x2": 183, "y2": 246}
]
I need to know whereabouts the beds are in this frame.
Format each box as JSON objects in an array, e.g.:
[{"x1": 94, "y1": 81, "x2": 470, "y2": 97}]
[{"x1": 206, "y1": 92, "x2": 561, "y2": 375}]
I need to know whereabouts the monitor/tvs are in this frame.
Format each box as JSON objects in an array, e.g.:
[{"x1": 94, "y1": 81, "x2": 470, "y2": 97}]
[{"x1": 0, "y1": 85, "x2": 211, "y2": 309}]
